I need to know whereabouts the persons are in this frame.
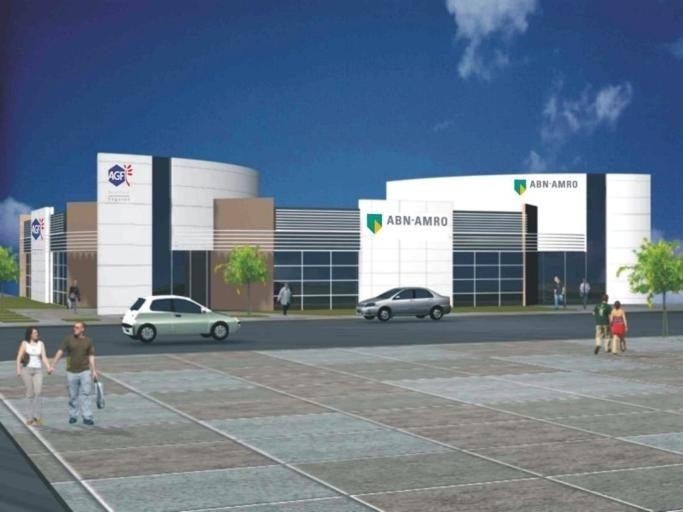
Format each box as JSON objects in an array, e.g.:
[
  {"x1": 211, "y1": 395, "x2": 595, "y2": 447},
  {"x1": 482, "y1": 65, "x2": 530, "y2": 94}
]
[
  {"x1": 579, "y1": 278, "x2": 590, "y2": 305},
  {"x1": 592, "y1": 292, "x2": 612, "y2": 355},
  {"x1": 276, "y1": 283, "x2": 292, "y2": 315},
  {"x1": 68, "y1": 279, "x2": 80, "y2": 313},
  {"x1": 15, "y1": 326, "x2": 49, "y2": 425},
  {"x1": 608, "y1": 301, "x2": 628, "y2": 354},
  {"x1": 554, "y1": 276, "x2": 565, "y2": 308},
  {"x1": 49, "y1": 321, "x2": 99, "y2": 424}
]
